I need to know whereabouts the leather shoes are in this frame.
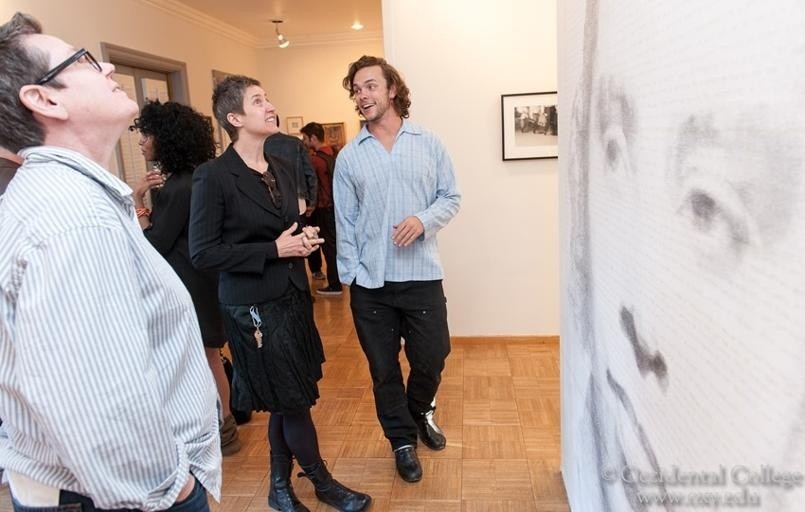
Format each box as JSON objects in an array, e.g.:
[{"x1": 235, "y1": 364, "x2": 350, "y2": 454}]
[
  {"x1": 395, "y1": 445, "x2": 420, "y2": 483},
  {"x1": 415, "y1": 408, "x2": 446, "y2": 452}
]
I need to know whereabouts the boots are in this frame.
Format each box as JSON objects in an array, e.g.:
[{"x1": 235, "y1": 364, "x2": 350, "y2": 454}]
[
  {"x1": 217, "y1": 412, "x2": 243, "y2": 457},
  {"x1": 300, "y1": 459, "x2": 373, "y2": 509},
  {"x1": 266, "y1": 450, "x2": 308, "y2": 512}
]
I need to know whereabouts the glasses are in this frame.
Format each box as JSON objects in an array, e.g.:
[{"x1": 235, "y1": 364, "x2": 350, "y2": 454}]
[
  {"x1": 35, "y1": 47, "x2": 102, "y2": 83},
  {"x1": 69, "y1": 85, "x2": 70, "y2": 86}
]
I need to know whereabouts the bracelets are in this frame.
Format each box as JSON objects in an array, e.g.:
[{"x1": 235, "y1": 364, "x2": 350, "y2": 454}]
[{"x1": 136, "y1": 208, "x2": 150, "y2": 218}]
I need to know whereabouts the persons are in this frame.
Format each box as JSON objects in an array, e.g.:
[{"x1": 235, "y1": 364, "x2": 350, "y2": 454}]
[
  {"x1": 127, "y1": 100, "x2": 242, "y2": 456},
  {"x1": 0, "y1": 12, "x2": 223, "y2": 512},
  {"x1": 332, "y1": 56, "x2": 462, "y2": 482},
  {"x1": 0, "y1": 146, "x2": 25, "y2": 195},
  {"x1": 188, "y1": 75, "x2": 371, "y2": 512},
  {"x1": 264, "y1": 114, "x2": 344, "y2": 295}
]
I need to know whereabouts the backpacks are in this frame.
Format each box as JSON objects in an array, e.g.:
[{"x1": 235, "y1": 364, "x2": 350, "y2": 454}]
[{"x1": 316, "y1": 147, "x2": 339, "y2": 182}]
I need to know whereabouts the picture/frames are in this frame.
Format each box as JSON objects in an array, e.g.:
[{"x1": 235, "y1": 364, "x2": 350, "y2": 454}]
[
  {"x1": 286, "y1": 115, "x2": 304, "y2": 135},
  {"x1": 500, "y1": 90, "x2": 558, "y2": 161},
  {"x1": 320, "y1": 122, "x2": 346, "y2": 156}
]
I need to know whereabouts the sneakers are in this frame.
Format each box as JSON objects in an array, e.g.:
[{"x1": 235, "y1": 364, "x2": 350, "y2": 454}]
[
  {"x1": 317, "y1": 284, "x2": 344, "y2": 296},
  {"x1": 311, "y1": 270, "x2": 326, "y2": 280}
]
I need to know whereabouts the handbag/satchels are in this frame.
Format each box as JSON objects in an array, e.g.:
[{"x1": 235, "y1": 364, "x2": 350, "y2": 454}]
[{"x1": 218, "y1": 354, "x2": 253, "y2": 426}]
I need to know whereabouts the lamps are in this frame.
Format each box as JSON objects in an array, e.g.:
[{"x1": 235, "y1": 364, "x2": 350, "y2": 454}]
[{"x1": 271, "y1": 20, "x2": 290, "y2": 48}]
